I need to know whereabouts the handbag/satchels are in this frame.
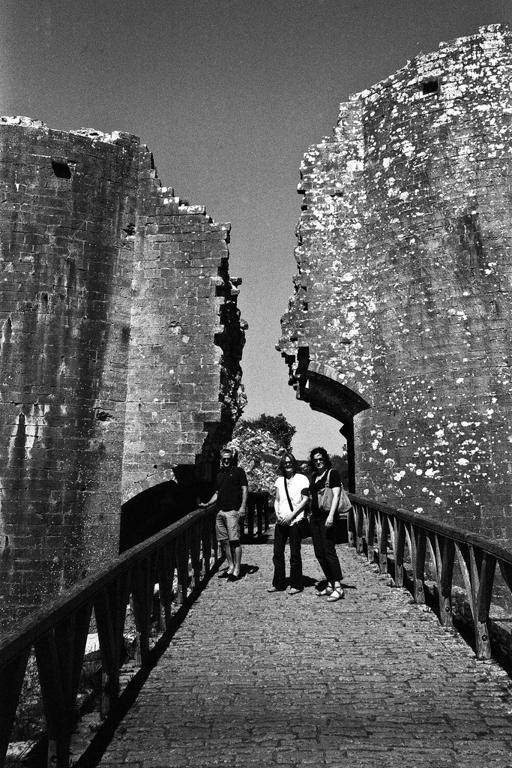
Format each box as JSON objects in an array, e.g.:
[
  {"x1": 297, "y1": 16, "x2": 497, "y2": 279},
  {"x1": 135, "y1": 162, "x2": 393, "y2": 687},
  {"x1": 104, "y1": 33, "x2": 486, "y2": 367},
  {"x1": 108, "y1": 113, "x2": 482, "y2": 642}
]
[
  {"x1": 317, "y1": 486, "x2": 353, "y2": 513},
  {"x1": 295, "y1": 518, "x2": 313, "y2": 539}
]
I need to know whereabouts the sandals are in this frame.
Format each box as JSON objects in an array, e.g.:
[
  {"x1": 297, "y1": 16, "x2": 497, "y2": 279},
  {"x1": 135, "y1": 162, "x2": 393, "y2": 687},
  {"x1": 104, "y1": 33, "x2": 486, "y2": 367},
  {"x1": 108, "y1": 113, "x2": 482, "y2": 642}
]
[{"x1": 316, "y1": 585, "x2": 344, "y2": 602}]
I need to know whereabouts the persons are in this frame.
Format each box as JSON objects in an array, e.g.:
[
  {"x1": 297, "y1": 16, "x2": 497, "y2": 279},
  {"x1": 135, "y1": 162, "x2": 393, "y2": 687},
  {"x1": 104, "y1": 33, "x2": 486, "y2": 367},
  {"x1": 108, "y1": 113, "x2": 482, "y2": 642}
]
[
  {"x1": 265, "y1": 450, "x2": 311, "y2": 597},
  {"x1": 196, "y1": 448, "x2": 250, "y2": 583},
  {"x1": 304, "y1": 446, "x2": 351, "y2": 603}
]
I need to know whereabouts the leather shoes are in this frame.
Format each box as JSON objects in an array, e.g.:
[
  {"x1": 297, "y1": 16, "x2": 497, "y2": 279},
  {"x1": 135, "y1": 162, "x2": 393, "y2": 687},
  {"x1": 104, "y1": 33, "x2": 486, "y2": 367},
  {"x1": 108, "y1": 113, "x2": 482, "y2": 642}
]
[
  {"x1": 218, "y1": 570, "x2": 242, "y2": 582},
  {"x1": 288, "y1": 588, "x2": 300, "y2": 594},
  {"x1": 267, "y1": 586, "x2": 284, "y2": 592}
]
